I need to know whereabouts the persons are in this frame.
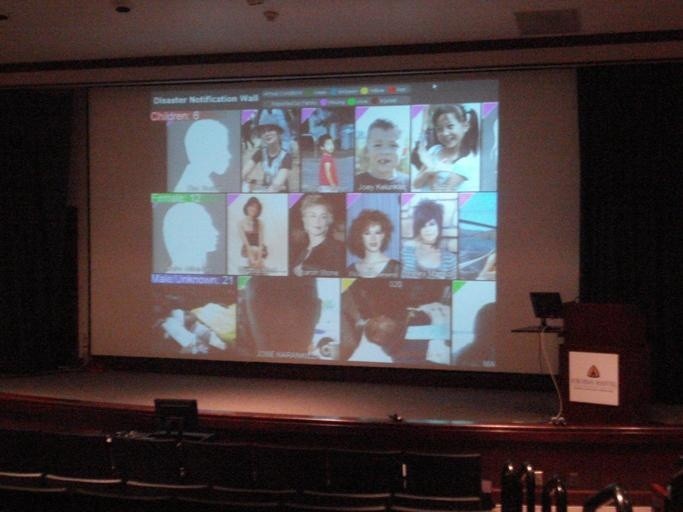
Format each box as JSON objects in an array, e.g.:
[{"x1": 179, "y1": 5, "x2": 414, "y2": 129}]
[{"x1": 236, "y1": 103, "x2": 499, "y2": 368}]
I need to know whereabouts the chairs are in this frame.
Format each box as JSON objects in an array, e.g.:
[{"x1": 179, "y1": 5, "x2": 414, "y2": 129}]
[{"x1": 0, "y1": 426, "x2": 635, "y2": 512}]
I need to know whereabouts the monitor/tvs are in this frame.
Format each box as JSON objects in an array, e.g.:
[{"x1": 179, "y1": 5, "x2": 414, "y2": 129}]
[{"x1": 147, "y1": 398, "x2": 202, "y2": 441}]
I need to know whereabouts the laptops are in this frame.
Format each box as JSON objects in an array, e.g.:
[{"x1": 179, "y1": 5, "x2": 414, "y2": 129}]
[{"x1": 528, "y1": 292, "x2": 568, "y2": 318}]
